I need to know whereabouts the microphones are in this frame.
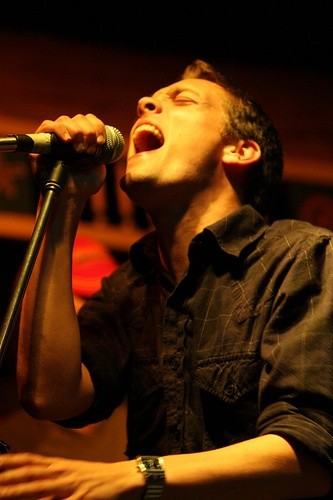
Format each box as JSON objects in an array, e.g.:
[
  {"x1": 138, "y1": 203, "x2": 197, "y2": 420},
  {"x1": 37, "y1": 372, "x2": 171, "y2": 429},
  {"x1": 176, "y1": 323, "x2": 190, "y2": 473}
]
[{"x1": 0, "y1": 125, "x2": 126, "y2": 164}]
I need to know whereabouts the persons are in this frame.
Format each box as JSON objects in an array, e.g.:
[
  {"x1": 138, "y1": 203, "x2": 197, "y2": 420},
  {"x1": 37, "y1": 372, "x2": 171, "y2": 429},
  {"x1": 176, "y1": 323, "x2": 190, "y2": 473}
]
[{"x1": 1, "y1": 60, "x2": 332, "y2": 500}]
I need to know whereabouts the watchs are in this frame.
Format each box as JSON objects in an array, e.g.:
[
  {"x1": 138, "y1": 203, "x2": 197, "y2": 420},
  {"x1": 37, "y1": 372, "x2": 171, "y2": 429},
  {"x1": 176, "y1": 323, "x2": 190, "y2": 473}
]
[{"x1": 136, "y1": 455, "x2": 167, "y2": 498}]
